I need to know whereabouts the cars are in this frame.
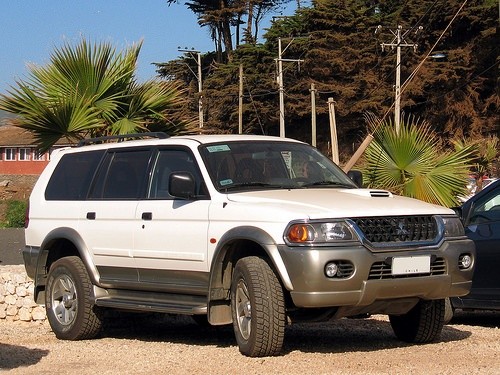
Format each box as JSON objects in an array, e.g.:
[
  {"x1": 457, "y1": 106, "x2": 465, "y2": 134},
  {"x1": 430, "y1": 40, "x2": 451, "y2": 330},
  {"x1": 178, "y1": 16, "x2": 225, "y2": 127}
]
[{"x1": 441, "y1": 177, "x2": 500, "y2": 326}]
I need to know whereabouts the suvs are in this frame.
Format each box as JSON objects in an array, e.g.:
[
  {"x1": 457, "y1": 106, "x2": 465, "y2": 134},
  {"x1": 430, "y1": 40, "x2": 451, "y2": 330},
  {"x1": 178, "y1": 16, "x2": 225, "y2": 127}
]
[{"x1": 20, "y1": 130, "x2": 478, "y2": 359}]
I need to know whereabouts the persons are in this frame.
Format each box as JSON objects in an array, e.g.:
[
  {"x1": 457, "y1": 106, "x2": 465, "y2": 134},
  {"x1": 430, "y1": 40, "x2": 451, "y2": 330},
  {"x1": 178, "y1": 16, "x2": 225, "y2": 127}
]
[{"x1": 301, "y1": 161, "x2": 325, "y2": 184}]
